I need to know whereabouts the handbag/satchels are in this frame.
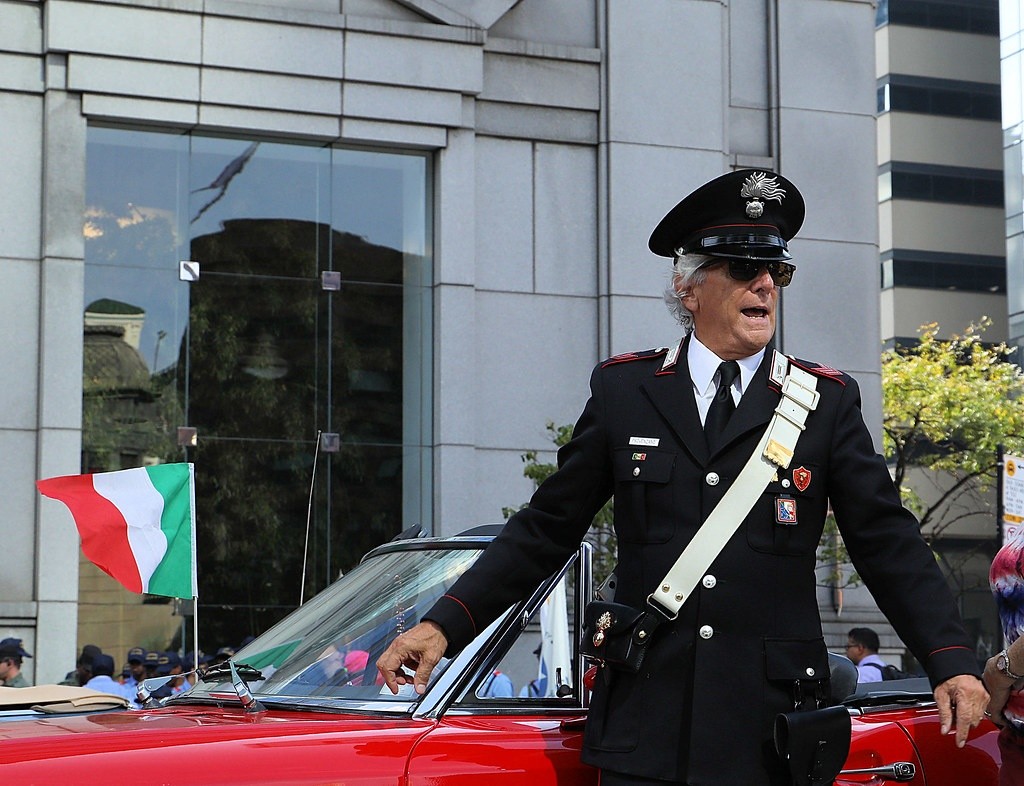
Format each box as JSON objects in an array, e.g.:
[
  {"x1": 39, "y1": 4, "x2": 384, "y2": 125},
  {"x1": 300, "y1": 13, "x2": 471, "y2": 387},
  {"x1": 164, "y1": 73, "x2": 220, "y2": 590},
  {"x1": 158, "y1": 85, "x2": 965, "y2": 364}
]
[{"x1": 578, "y1": 601, "x2": 656, "y2": 675}]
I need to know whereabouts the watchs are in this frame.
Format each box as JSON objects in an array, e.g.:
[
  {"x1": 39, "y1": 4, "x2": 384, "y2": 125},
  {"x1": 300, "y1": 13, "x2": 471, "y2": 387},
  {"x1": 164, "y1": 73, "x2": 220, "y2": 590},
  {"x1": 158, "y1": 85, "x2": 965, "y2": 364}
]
[{"x1": 997, "y1": 648, "x2": 1024, "y2": 683}]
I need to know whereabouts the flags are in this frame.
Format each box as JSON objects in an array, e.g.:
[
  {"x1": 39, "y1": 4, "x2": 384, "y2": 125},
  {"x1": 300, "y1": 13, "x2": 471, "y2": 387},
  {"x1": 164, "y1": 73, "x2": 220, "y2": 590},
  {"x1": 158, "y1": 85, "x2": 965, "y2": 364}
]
[
  {"x1": 540, "y1": 575, "x2": 573, "y2": 699},
  {"x1": 36, "y1": 462, "x2": 199, "y2": 599}
]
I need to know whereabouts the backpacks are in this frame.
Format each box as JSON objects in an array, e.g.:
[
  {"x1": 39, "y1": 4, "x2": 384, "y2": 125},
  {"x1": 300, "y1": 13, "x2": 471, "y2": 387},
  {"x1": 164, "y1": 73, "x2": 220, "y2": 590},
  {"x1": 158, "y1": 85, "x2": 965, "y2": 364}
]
[{"x1": 862, "y1": 663, "x2": 918, "y2": 681}]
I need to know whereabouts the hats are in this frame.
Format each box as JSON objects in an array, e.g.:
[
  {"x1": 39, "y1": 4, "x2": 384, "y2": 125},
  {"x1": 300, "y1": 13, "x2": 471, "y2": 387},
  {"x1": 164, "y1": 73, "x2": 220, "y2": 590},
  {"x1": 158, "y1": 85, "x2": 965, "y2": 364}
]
[
  {"x1": 648, "y1": 168, "x2": 807, "y2": 260},
  {"x1": 0, "y1": 638, "x2": 33, "y2": 659},
  {"x1": 73, "y1": 642, "x2": 235, "y2": 676},
  {"x1": 533, "y1": 642, "x2": 543, "y2": 654}
]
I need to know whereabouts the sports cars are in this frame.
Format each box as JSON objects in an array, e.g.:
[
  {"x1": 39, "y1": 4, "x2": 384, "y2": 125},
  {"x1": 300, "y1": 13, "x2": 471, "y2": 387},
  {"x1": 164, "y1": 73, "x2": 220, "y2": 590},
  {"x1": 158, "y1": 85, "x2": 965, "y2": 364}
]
[{"x1": 0, "y1": 531, "x2": 1023, "y2": 786}]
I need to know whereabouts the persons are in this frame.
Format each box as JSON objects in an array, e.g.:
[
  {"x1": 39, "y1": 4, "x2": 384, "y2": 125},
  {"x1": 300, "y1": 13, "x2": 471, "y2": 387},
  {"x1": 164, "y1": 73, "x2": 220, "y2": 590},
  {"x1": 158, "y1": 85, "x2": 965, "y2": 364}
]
[
  {"x1": 0, "y1": 638, "x2": 544, "y2": 712},
  {"x1": 376, "y1": 168, "x2": 990, "y2": 786},
  {"x1": 845, "y1": 627, "x2": 888, "y2": 683},
  {"x1": 982, "y1": 521, "x2": 1024, "y2": 786}
]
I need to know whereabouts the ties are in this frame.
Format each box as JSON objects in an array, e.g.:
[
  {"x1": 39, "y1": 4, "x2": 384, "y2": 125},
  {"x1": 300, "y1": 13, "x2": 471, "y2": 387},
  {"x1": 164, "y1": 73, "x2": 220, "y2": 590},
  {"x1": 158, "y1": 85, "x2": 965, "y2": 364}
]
[{"x1": 704, "y1": 362, "x2": 741, "y2": 453}]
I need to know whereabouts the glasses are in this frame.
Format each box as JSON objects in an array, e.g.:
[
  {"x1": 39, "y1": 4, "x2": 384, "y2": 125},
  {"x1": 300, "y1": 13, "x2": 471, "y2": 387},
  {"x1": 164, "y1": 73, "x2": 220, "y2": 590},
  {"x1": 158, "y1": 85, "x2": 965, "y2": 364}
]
[
  {"x1": 698, "y1": 255, "x2": 796, "y2": 287},
  {"x1": 844, "y1": 643, "x2": 865, "y2": 651}
]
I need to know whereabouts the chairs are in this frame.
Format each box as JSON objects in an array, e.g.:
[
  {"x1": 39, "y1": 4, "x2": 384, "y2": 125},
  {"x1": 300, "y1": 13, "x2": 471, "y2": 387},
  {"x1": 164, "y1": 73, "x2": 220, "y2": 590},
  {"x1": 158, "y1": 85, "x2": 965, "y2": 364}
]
[{"x1": 825, "y1": 653, "x2": 858, "y2": 702}]
